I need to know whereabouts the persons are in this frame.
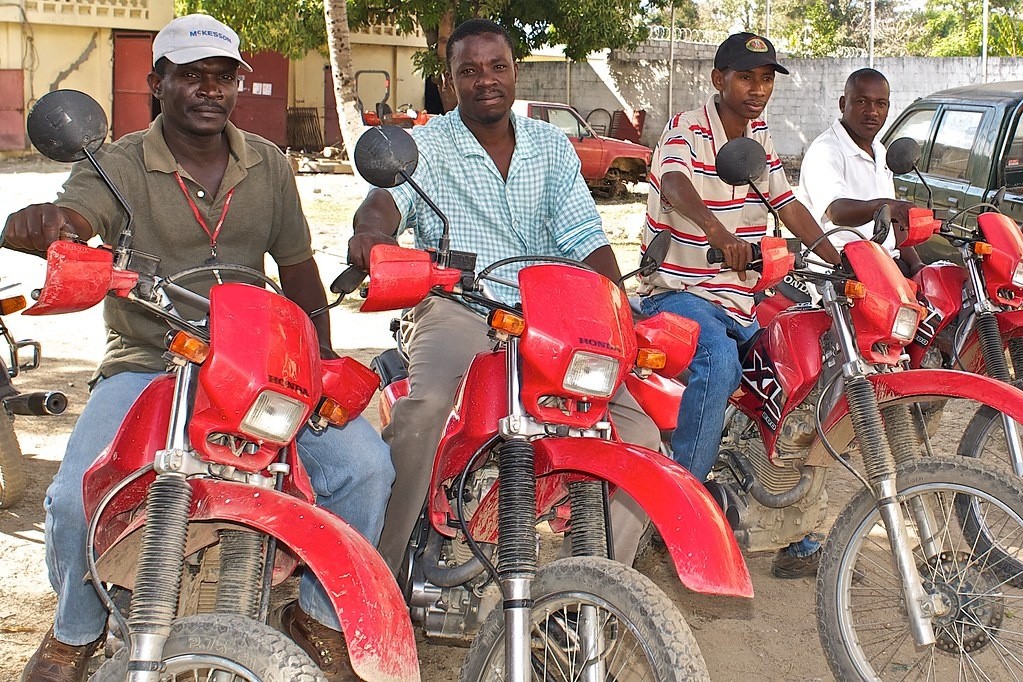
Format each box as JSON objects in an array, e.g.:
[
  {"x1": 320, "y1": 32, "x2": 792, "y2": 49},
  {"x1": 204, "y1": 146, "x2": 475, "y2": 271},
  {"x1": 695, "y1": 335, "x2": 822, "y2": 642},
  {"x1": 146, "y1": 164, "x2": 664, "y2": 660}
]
[
  {"x1": 637, "y1": 32, "x2": 865, "y2": 587},
  {"x1": 796, "y1": 69, "x2": 926, "y2": 278},
  {"x1": 2, "y1": 16, "x2": 397, "y2": 682},
  {"x1": 345, "y1": 17, "x2": 661, "y2": 682}
]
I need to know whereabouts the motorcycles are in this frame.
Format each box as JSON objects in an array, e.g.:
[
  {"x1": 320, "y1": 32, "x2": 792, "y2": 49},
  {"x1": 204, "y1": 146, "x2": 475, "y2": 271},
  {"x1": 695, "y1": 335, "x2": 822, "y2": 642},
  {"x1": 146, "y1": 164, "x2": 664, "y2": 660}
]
[{"x1": 0, "y1": 90, "x2": 1023, "y2": 682}]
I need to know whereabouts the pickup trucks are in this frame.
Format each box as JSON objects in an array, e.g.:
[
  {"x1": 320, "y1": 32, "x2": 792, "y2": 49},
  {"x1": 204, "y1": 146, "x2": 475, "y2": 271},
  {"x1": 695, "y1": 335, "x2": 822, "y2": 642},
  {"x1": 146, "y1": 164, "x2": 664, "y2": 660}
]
[
  {"x1": 508, "y1": 99, "x2": 653, "y2": 200},
  {"x1": 881, "y1": 79, "x2": 1023, "y2": 269}
]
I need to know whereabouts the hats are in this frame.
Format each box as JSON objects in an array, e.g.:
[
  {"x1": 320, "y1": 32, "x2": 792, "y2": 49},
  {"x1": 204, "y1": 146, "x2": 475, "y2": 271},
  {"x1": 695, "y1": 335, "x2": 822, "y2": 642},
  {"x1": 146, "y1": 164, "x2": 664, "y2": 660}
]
[
  {"x1": 152, "y1": 13, "x2": 253, "y2": 73},
  {"x1": 714, "y1": 32, "x2": 789, "y2": 74}
]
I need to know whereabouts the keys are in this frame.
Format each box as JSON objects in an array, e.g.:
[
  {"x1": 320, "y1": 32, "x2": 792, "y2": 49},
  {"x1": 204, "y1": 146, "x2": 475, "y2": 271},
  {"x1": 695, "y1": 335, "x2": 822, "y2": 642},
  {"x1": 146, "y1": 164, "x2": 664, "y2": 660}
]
[{"x1": 204, "y1": 255, "x2": 222, "y2": 285}]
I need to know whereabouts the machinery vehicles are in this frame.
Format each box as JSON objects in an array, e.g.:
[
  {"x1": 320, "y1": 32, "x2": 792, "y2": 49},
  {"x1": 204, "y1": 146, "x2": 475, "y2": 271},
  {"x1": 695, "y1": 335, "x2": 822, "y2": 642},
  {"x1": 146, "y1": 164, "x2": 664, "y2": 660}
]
[{"x1": 353, "y1": 71, "x2": 444, "y2": 126}]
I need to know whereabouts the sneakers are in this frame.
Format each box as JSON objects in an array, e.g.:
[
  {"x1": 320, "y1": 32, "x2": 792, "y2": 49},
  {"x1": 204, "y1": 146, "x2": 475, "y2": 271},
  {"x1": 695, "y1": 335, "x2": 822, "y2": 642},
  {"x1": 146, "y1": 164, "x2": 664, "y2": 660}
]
[
  {"x1": 269, "y1": 598, "x2": 367, "y2": 682},
  {"x1": 22, "y1": 607, "x2": 111, "y2": 682},
  {"x1": 771, "y1": 546, "x2": 864, "y2": 584}
]
